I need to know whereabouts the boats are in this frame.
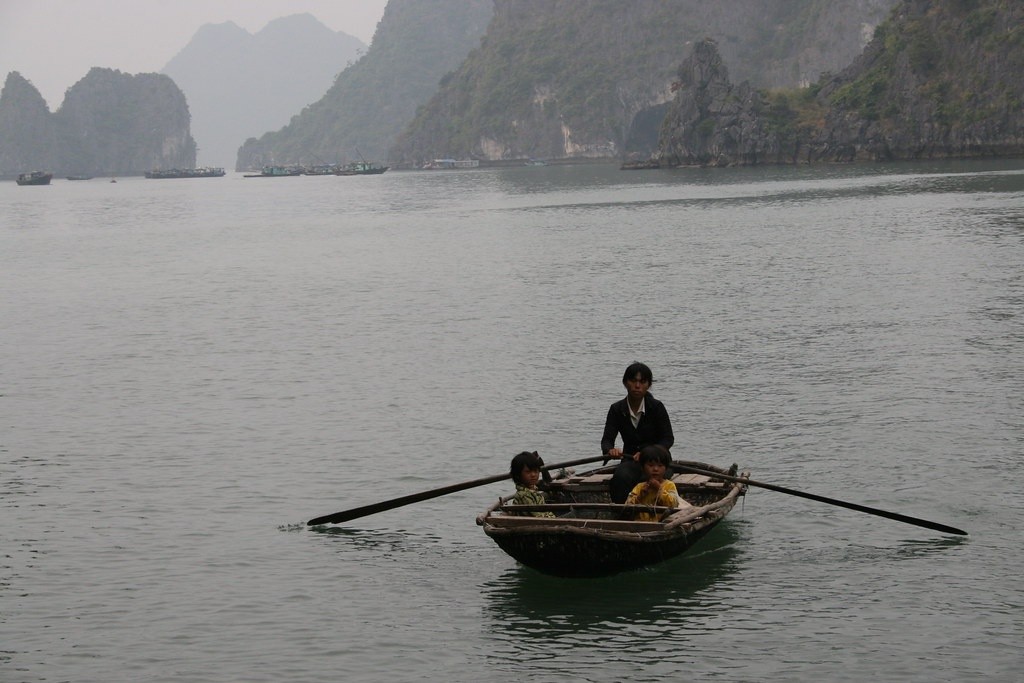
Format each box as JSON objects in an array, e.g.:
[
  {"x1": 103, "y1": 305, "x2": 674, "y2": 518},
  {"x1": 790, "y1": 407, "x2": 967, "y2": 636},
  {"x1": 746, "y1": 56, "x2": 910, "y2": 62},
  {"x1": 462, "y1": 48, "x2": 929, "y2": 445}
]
[
  {"x1": 244, "y1": 149, "x2": 390, "y2": 177},
  {"x1": 476, "y1": 458, "x2": 751, "y2": 578},
  {"x1": 16, "y1": 171, "x2": 53, "y2": 186},
  {"x1": 65, "y1": 175, "x2": 93, "y2": 180},
  {"x1": 144, "y1": 167, "x2": 226, "y2": 178}
]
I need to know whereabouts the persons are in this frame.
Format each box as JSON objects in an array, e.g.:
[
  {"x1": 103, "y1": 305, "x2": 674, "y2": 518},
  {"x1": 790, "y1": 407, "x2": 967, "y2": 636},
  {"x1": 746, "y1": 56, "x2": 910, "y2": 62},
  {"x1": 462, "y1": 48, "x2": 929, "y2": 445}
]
[
  {"x1": 624, "y1": 444, "x2": 679, "y2": 522},
  {"x1": 508, "y1": 451, "x2": 556, "y2": 519},
  {"x1": 601, "y1": 363, "x2": 674, "y2": 499}
]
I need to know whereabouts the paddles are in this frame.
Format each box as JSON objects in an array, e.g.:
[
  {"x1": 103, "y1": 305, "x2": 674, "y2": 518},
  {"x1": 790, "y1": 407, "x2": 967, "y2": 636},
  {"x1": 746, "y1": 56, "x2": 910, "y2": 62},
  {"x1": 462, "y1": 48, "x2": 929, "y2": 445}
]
[
  {"x1": 625, "y1": 450, "x2": 969, "y2": 537},
  {"x1": 305, "y1": 453, "x2": 625, "y2": 528}
]
